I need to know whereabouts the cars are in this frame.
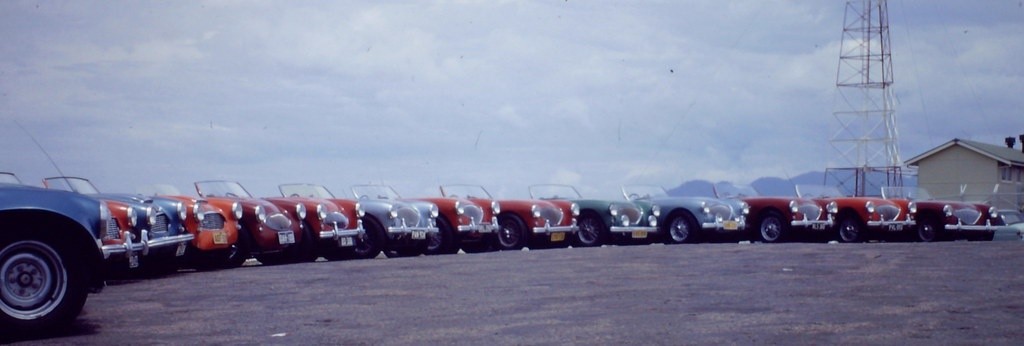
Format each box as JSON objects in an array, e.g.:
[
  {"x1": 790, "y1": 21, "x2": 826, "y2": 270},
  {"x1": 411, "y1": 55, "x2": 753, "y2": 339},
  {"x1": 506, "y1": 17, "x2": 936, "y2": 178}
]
[
  {"x1": 195, "y1": 180, "x2": 366, "y2": 264},
  {"x1": 440, "y1": 183, "x2": 580, "y2": 251},
  {"x1": 102, "y1": 200, "x2": 139, "y2": 287},
  {"x1": 0, "y1": 183, "x2": 110, "y2": 339},
  {"x1": 745, "y1": 185, "x2": 919, "y2": 242},
  {"x1": 279, "y1": 183, "x2": 443, "y2": 259},
  {"x1": 880, "y1": 186, "x2": 1020, "y2": 242},
  {"x1": 144, "y1": 184, "x2": 307, "y2": 268},
  {"x1": 352, "y1": 183, "x2": 502, "y2": 257},
  {"x1": 529, "y1": 185, "x2": 660, "y2": 247},
  {"x1": 622, "y1": 183, "x2": 748, "y2": 245},
  {"x1": 712, "y1": 182, "x2": 839, "y2": 243},
  {"x1": 42, "y1": 176, "x2": 245, "y2": 270},
  {"x1": 0, "y1": 172, "x2": 190, "y2": 275}
]
